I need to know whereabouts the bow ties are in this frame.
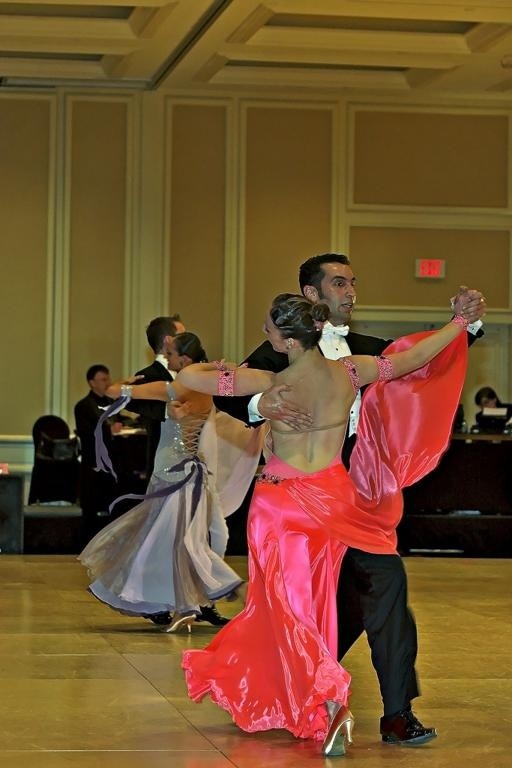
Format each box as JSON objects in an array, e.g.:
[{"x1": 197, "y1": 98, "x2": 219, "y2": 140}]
[{"x1": 322, "y1": 321, "x2": 349, "y2": 336}]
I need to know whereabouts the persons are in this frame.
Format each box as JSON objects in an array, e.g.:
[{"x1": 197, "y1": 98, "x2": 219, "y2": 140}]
[
  {"x1": 123, "y1": 313, "x2": 233, "y2": 627},
  {"x1": 77, "y1": 331, "x2": 265, "y2": 633},
  {"x1": 212, "y1": 252, "x2": 487, "y2": 745},
  {"x1": 74, "y1": 364, "x2": 149, "y2": 517},
  {"x1": 474, "y1": 386, "x2": 512, "y2": 436},
  {"x1": 176, "y1": 288, "x2": 487, "y2": 756}
]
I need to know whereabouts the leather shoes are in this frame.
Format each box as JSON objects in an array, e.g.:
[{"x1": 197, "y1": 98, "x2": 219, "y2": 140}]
[
  {"x1": 140, "y1": 611, "x2": 173, "y2": 625},
  {"x1": 379, "y1": 708, "x2": 436, "y2": 746},
  {"x1": 195, "y1": 604, "x2": 231, "y2": 625}
]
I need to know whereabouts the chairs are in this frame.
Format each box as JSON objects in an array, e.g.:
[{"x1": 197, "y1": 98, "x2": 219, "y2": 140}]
[{"x1": 18, "y1": 414, "x2": 86, "y2": 555}]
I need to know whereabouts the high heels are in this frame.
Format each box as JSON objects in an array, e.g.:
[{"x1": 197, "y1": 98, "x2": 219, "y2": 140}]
[
  {"x1": 321, "y1": 704, "x2": 356, "y2": 756},
  {"x1": 160, "y1": 613, "x2": 197, "y2": 633}
]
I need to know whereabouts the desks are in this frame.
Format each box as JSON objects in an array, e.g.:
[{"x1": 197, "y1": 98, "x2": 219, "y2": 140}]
[{"x1": 395, "y1": 435, "x2": 512, "y2": 556}]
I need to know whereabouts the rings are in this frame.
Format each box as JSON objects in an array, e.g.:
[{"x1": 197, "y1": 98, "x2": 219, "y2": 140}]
[{"x1": 479, "y1": 298, "x2": 485, "y2": 303}]
